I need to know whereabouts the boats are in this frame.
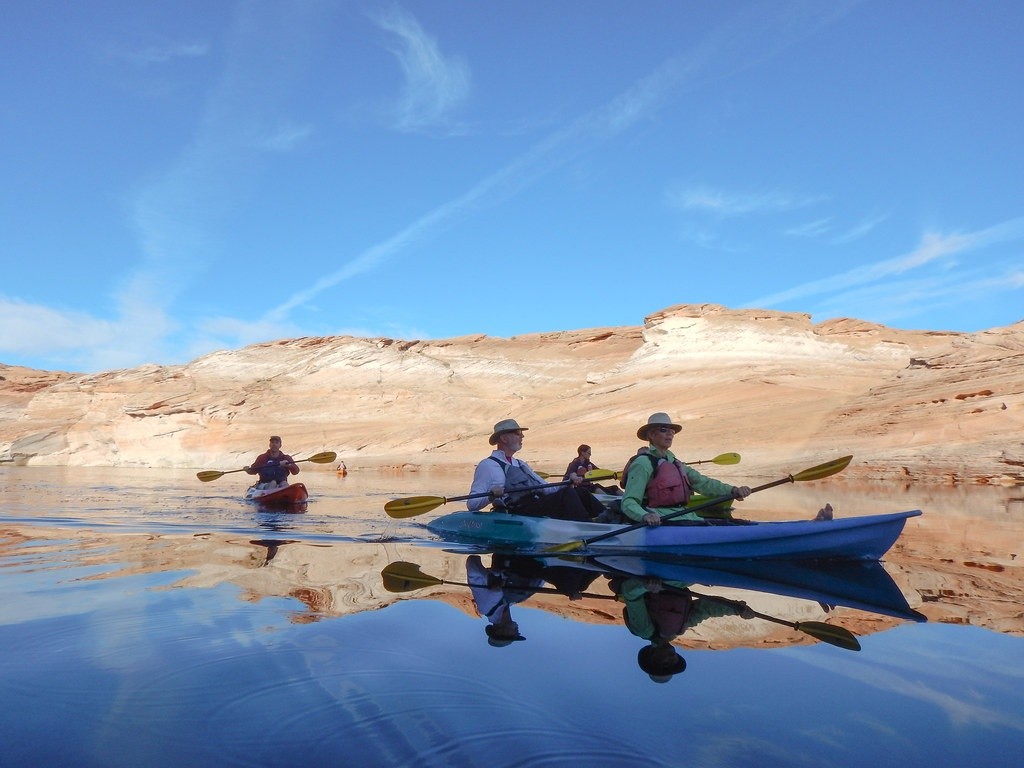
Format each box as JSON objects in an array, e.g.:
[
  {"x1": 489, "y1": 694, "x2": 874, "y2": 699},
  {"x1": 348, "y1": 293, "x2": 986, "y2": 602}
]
[
  {"x1": 338, "y1": 469, "x2": 346, "y2": 474},
  {"x1": 427, "y1": 507, "x2": 923, "y2": 566},
  {"x1": 245, "y1": 482, "x2": 308, "y2": 504},
  {"x1": 486, "y1": 551, "x2": 928, "y2": 623}
]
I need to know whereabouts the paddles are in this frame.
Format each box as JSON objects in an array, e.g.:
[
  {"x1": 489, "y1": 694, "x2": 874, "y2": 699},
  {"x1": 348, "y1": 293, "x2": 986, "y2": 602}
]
[
  {"x1": 380, "y1": 561, "x2": 626, "y2": 604},
  {"x1": 540, "y1": 453, "x2": 854, "y2": 554},
  {"x1": 534, "y1": 453, "x2": 741, "y2": 480},
  {"x1": 661, "y1": 582, "x2": 862, "y2": 653},
  {"x1": 384, "y1": 470, "x2": 624, "y2": 520},
  {"x1": 197, "y1": 452, "x2": 338, "y2": 482}
]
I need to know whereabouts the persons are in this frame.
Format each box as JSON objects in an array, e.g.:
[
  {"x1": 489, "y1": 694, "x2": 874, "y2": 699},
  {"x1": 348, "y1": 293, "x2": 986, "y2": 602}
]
[
  {"x1": 337, "y1": 461, "x2": 346, "y2": 471},
  {"x1": 621, "y1": 577, "x2": 755, "y2": 683},
  {"x1": 620, "y1": 413, "x2": 833, "y2": 526},
  {"x1": 466, "y1": 419, "x2": 607, "y2": 522},
  {"x1": 562, "y1": 444, "x2": 624, "y2": 496},
  {"x1": 465, "y1": 553, "x2": 582, "y2": 647},
  {"x1": 243, "y1": 436, "x2": 300, "y2": 490}
]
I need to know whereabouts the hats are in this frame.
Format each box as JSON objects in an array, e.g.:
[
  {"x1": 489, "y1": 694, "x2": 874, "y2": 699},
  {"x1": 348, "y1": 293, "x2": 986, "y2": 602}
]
[
  {"x1": 485, "y1": 624, "x2": 526, "y2": 648},
  {"x1": 270, "y1": 436, "x2": 281, "y2": 440},
  {"x1": 638, "y1": 645, "x2": 687, "y2": 684},
  {"x1": 489, "y1": 419, "x2": 529, "y2": 446},
  {"x1": 637, "y1": 412, "x2": 682, "y2": 440}
]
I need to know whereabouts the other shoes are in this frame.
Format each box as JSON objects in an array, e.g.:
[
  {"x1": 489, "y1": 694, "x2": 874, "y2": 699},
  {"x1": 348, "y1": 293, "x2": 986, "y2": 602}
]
[
  {"x1": 266, "y1": 481, "x2": 277, "y2": 489},
  {"x1": 279, "y1": 481, "x2": 289, "y2": 489}
]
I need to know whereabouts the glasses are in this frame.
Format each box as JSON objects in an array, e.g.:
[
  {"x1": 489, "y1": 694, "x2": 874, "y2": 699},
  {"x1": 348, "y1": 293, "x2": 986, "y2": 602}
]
[{"x1": 656, "y1": 427, "x2": 677, "y2": 433}]
